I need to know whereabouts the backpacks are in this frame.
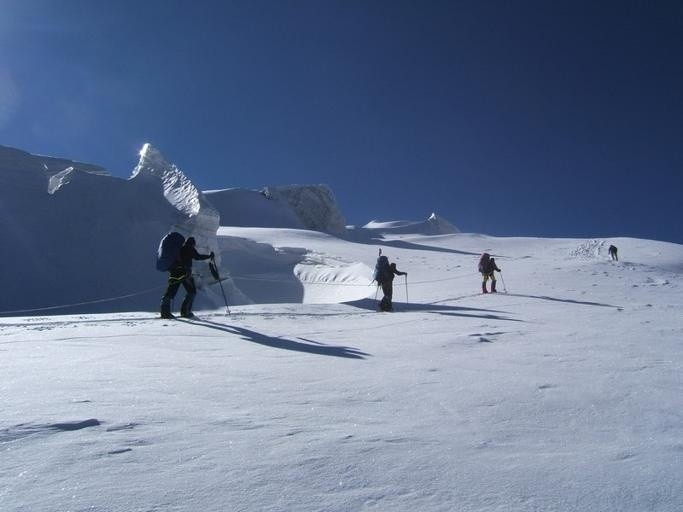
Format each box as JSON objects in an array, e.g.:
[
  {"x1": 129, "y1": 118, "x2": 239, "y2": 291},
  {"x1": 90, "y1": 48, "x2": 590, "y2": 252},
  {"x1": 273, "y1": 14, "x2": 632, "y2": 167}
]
[
  {"x1": 372, "y1": 254, "x2": 389, "y2": 283},
  {"x1": 154, "y1": 230, "x2": 184, "y2": 271},
  {"x1": 476, "y1": 252, "x2": 491, "y2": 273}
]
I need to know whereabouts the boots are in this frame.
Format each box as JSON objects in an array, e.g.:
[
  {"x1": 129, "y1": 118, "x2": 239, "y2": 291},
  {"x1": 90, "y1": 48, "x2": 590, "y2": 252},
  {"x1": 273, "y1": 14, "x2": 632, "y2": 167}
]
[
  {"x1": 158, "y1": 292, "x2": 196, "y2": 320},
  {"x1": 482, "y1": 282, "x2": 489, "y2": 293},
  {"x1": 490, "y1": 280, "x2": 497, "y2": 293}
]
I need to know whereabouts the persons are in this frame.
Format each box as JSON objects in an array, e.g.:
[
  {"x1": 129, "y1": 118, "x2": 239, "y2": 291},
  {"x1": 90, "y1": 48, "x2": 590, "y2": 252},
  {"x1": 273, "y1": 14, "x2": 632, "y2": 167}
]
[
  {"x1": 379, "y1": 260, "x2": 408, "y2": 311},
  {"x1": 482, "y1": 257, "x2": 501, "y2": 293},
  {"x1": 160, "y1": 236, "x2": 214, "y2": 319},
  {"x1": 608, "y1": 245, "x2": 618, "y2": 261}
]
